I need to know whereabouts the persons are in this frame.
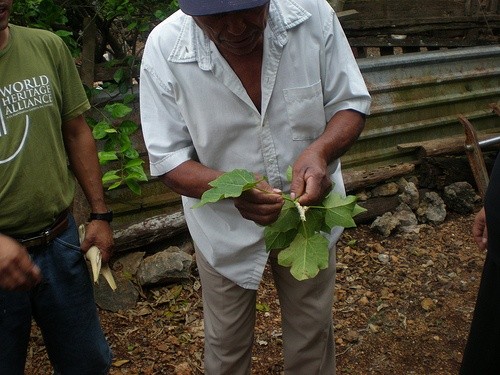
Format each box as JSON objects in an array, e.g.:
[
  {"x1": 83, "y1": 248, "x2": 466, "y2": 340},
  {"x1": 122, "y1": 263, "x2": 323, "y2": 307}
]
[
  {"x1": 0, "y1": 0, "x2": 113, "y2": 375},
  {"x1": 140, "y1": 0, "x2": 371, "y2": 375}
]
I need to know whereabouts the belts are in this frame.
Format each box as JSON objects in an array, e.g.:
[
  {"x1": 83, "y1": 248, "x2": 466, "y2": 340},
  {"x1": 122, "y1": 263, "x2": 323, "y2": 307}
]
[{"x1": 20, "y1": 212, "x2": 69, "y2": 249}]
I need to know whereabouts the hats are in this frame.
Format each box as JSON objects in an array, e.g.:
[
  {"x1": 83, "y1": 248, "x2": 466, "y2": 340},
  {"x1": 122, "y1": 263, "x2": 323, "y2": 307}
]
[{"x1": 180, "y1": 0, "x2": 270, "y2": 15}]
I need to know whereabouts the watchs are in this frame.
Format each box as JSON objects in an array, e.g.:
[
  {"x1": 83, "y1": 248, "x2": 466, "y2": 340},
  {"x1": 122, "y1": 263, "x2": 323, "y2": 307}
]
[{"x1": 88, "y1": 209, "x2": 113, "y2": 225}]
